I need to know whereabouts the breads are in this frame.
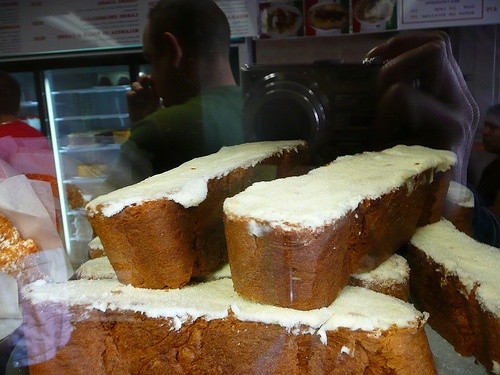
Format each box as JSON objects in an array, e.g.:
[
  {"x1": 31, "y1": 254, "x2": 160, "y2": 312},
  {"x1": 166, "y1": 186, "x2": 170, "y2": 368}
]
[{"x1": 21, "y1": 140, "x2": 500, "y2": 375}]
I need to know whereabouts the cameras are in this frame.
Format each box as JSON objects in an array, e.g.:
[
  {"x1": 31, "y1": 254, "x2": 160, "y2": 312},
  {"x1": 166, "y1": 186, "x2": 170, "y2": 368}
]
[{"x1": 240, "y1": 56, "x2": 390, "y2": 165}]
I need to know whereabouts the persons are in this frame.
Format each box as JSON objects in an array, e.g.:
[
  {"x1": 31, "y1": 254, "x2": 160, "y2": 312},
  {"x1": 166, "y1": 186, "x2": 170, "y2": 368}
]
[
  {"x1": 90, "y1": 0, "x2": 254, "y2": 203},
  {"x1": 0, "y1": 70, "x2": 65, "y2": 251},
  {"x1": 474, "y1": 105, "x2": 500, "y2": 250}
]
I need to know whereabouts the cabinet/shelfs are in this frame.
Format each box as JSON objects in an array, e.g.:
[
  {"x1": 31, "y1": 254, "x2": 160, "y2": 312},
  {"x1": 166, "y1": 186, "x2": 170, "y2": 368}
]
[{"x1": 0, "y1": 36, "x2": 253, "y2": 268}]
